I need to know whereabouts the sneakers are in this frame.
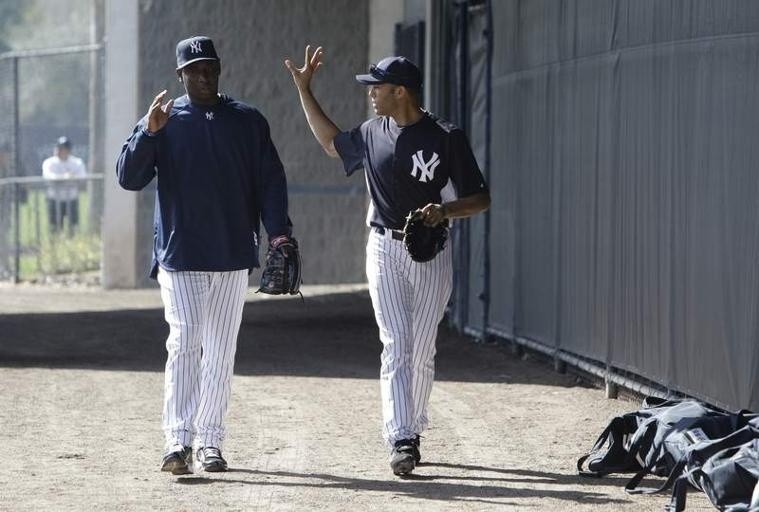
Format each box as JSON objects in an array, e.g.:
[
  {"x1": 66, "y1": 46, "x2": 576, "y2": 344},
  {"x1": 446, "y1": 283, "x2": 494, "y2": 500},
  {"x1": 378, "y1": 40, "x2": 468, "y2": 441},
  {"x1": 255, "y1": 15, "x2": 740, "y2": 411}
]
[
  {"x1": 195, "y1": 447, "x2": 227, "y2": 472},
  {"x1": 161, "y1": 447, "x2": 194, "y2": 475},
  {"x1": 391, "y1": 433, "x2": 420, "y2": 475}
]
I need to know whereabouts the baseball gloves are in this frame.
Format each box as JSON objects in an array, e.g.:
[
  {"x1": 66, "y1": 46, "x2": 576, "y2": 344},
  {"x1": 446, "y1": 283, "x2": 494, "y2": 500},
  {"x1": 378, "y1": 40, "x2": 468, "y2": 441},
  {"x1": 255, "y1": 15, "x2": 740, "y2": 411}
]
[
  {"x1": 402, "y1": 211, "x2": 449, "y2": 263},
  {"x1": 259, "y1": 235, "x2": 301, "y2": 295}
]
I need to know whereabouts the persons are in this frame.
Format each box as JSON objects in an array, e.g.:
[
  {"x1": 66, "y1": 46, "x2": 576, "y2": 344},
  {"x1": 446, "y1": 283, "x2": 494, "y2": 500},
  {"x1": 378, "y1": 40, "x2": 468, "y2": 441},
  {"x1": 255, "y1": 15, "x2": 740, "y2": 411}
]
[
  {"x1": 41, "y1": 135, "x2": 88, "y2": 240},
  {"x1": 0, "y1": 132, "x2": 32, "y2": 281},
  {"x1": 115, "y1": 35, "x2": 302, "y2": 478},
  {"x1": 284, "y1": 39, "x2": 492, "y2": 480}
]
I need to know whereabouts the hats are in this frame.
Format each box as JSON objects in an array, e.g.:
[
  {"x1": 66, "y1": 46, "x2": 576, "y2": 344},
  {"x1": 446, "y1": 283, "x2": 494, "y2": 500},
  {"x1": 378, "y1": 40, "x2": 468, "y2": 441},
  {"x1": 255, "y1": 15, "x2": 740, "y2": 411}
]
[
  {"x1": 58, "y1": 135, "x2": 70, "y2": 145},
  {"x1": 176, "y1": 36, "x2": 219, "y2": 70},
  {"x1": 354, "y1": 56, "x2": 423, "y2": 88}
]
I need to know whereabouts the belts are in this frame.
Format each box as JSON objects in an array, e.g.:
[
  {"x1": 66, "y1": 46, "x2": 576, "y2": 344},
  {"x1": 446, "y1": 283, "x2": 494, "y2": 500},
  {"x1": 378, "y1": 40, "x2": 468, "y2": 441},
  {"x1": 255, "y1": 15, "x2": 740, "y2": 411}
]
[{"x1": 375, "y1": 228, "x2": 404, "y2": 240}]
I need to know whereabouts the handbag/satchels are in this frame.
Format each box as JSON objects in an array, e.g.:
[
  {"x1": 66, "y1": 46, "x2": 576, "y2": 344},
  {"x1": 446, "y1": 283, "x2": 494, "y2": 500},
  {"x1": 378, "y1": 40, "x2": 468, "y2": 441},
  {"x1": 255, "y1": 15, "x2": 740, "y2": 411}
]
[{"x1": 589, "y1": 400, "x2": 759, "y2": 512}]
